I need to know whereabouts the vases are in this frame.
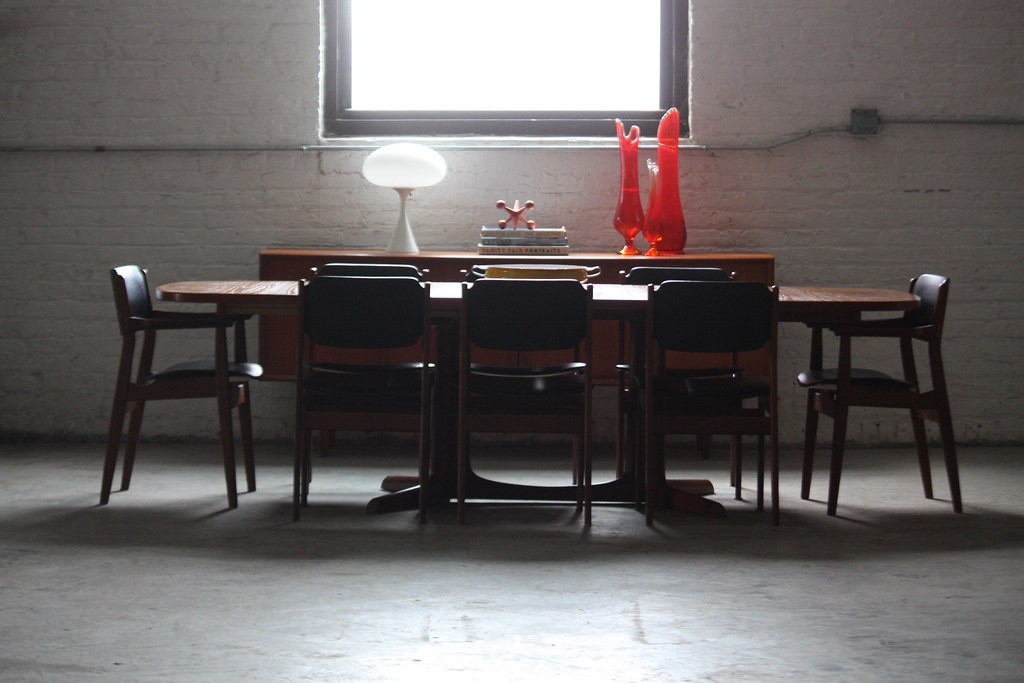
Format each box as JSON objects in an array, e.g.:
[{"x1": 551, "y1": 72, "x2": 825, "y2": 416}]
[{"x1": 614, "y1": 105, "x2": 688, "y2": 257}]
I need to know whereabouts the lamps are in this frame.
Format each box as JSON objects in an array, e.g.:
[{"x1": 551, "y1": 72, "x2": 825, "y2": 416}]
[{"x1": 362, "y1": 142, "x2": 446, "y2": 253}]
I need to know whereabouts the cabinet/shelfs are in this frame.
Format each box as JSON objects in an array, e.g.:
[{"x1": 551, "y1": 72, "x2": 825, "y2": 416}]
[{"x1": 258, "y1": 249, "x2": 775, "y2": 462}]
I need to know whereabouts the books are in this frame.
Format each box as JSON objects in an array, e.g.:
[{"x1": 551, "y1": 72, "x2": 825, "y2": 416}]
[{"x1": 477, "y1": 225, "x2": 570, "y2": 255}]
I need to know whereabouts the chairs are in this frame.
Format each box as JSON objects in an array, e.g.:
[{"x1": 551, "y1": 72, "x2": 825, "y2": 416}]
[
  {"x1": 797, "y1": 273, "x2": 962, "y2": 518},
  {"x1": 297, "y1": 264, "x2": 602, "y2": 526},
  {"x1": 97, "y1": 264, "x2": 264, "y2": 510},
  {"x1": 619, "y1": 265, "x2": 779, "y2": 527}
]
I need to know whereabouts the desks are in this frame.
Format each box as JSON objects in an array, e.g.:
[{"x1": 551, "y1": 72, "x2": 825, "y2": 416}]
[{"x1": 154, "y1": 279, "x2": 922, "y2": 519}]
